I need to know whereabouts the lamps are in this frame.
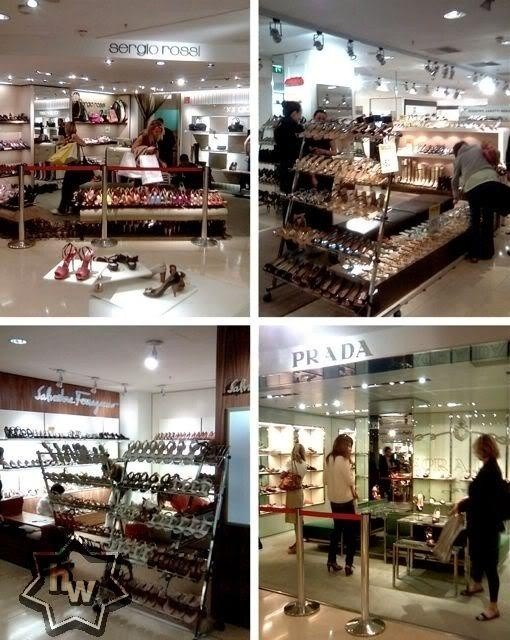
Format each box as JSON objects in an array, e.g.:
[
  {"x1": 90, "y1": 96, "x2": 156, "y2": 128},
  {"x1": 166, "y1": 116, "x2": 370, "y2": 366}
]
[
  {"x1": 268, "y1": 18, "x2": 510, "y2": 91},
  {"x1": 143, "y1": 340, "x2": 163, "y2": 370},
  {"x1": 52, "y1": 369, "x2": 168, "y2": 400}
]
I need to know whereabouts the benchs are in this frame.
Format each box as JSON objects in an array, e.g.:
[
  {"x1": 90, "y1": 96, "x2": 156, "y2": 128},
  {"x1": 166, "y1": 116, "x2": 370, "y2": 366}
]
[
  {"x1": 337, "y1": 194, "x2": 453, "y2": 240},
  {"x1": 80, "y1": 208, "x2": 226, "y2": 237}
]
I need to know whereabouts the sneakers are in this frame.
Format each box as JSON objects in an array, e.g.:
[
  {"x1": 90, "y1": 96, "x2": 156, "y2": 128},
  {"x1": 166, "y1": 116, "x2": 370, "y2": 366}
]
[{"x1": 50, "y1": 208, "x2": 67, "y2": 216}]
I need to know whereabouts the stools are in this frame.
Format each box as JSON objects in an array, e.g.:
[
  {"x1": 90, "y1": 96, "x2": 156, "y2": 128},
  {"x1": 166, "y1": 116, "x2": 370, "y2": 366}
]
[
  {"x1": 0, "y1": 512, "x2": 57, "y2": 570},
  {"x1": 393, "y1": 539, "x2": 463, "y2": 597},
  {"x1": 0, "y1": 205, "x2": 38, "y2": 236}
]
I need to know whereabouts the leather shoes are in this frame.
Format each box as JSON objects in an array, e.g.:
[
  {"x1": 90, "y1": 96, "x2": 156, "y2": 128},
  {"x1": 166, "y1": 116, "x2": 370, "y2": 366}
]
[
  {"x1": 312, "y1": 228, "x2": 378, "y2": 261},
  {"x1": 263, "y1": 253, "x2": 369, "y2": 312},
  {"x1": 2, "y1": 460, "x2": 46, "y2": 470},
  {"x1": 415, "y1": 493, "x2": 446, "y2": 548},
  {"x1": 1, "y1": 138, "x2": 30, "y2": 151},
  {"x1": 98, "y1": 433, "x2": 130, "y2": 441}
]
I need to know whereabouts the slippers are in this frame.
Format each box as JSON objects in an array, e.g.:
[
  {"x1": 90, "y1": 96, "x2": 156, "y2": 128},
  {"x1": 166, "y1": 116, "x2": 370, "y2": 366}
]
[
  {"x1": 460, "y1": 587, "x2": 483, "y2": 597},
  {"x1": 476, "y1": 613, "x2": 500, "y2": 623},
  {"x1": 132, "y1": 582, "x2": 201, "y2": 625}
]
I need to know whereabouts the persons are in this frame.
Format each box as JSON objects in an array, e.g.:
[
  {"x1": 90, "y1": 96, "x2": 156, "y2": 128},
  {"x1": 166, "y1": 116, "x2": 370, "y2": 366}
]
[
  {"x1": 131, "y1": 120, "x2": 167, "y2": 170},
  {"x1": 157, "y1": 118, "x2": 176, "y2": 167},
  {"x1": 451, "y1": 142, "x2": 510, "y2": 260},
  {"x1": 36, "y1": 484, "x2": 70, "y2": 518},
  {"x1": 34, "y1": 161, "x2": 55, "y2": 181},
  {"x1": 177, "y1": 155, "x2": 203, "y2": 189},
  {"x1": 325, "y1": 434, "x2": 358, "y2": 576},
  {"x1": 58, "y1": 118, "x2": 66, "y2": 135},
  {"x1": 305, "y1": 109, "x2": 337, "y2": 228},
  {"x1": 50, "y1": 162, "x2": 82, "y2": 216},
  {"x1": 379, "y1": 447, "x2": 393, "y2": 501},
  {"x1": 448, "y1": 434, "x2": 506, "y2": 621},
  {"x1": 157, "y1": 491, "x2": 208, "y2": 513},
  {"x1": 274, "y1": 101, "x2": 306, "y2": 251},
  {"x1": 281, "y1": 444, "x2": 307, "y2": 554},
  {"x1": 101, "y1": 461, "x2": 133, "y2": 536}
]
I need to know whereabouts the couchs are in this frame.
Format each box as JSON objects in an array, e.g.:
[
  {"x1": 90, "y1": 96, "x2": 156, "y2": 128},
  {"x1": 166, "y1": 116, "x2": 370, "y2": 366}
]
[
  {"x1": 303, "y1": 499, "x2": 396, "y2": 549},
  {"x1": 383, "y1": 503, "x2": 509, "y2": 572}
]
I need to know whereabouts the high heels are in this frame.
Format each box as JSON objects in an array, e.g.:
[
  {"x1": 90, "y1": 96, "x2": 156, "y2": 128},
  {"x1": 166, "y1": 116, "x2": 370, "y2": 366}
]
[
  {"x1": 345, "y1": 567, "x2": 353, "y2": 577},
  {"x1": 143, "y1": 272, "x2": 181, "y2": 299},
  {"x1": 54, "y1": 243, "x2": 78, "y2": 280},
  {"x1": 76, "y1": 246, "x2": 95, "y2": 282},
  {"x1": 327, "y1": 563, "x2": 343, "y2": 573}
]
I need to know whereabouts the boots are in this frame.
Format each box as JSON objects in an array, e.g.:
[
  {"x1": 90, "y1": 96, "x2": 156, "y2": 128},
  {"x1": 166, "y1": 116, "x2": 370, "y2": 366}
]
[{"x1": 34, "y1": 161, "x2": 51, "y2": 182}]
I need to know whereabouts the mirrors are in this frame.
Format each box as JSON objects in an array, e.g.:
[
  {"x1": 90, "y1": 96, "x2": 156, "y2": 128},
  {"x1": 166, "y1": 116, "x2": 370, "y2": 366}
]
[{"x1": 33, "y1": 98, "x2": 70, "y2": 188}]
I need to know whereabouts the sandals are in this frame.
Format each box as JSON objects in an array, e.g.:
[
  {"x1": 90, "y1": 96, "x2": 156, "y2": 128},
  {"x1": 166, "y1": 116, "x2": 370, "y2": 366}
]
[
  {"x1": 288, "y1": 548, "x2": 296, "y2": 555},
  {"x1": 121, "y1": 439, "x2": 210, "y2": 465},
  {"x1": 286, "y1": 188, "x2": 389, "y2": 221},
  {"x1": 119, "y1": 472, "x2": 213, "y2": 498},
  {"x1": 298, "y1": 119, "x2": 394, "y2": 142},
  {"x1": 0, "y1": 112, "x2": 28, "y2": 122},
  {"x1": 111, "y1": 501, "x2": 211, "y2": 541},
  {"x1": 3, "y1": 426, "x2": 98, "y2": 440},
  {"x1": 74, "y1": 186, "x2": 229, "y2": 209},
  {"x1": 108, "y1": 537, "x2": 206, "y2": 584},
  {"x1": 95, "y1": 253, "x2": 138, "y2": 272},
  {"x1": 289, "y1": 543, "x2": 297, "y2": 550},
  {"x1": 293, "y1": 154, "x2": 382, "y2": 184}
]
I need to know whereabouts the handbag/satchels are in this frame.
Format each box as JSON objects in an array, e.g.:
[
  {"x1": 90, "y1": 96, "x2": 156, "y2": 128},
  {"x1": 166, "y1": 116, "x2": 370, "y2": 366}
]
[
  {"x1": 47, "y1": 143, "x2": 73, "y2": 165},
  {"x1": 480, "y1": 140, "x2": 500, "y2": 167},
  {"x1": 80, "y1": 155, "x2": 95, "y2": 186},
  {"x1": 279, "y1": 461, "x2": 302, "y2": 492},
  {"x1": 64, "y1": 142, "x2": 78, "y2": 165},
  {"x1": 139, "y1": 153, "x2": 164, "y2": 185},
  {"x1": 72, "y1": 99, "x2": 126, "y2": 124}
]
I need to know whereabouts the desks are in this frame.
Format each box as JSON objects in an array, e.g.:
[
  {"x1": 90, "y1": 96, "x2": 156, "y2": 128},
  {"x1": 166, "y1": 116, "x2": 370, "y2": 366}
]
[{"x1": 396, "y1": 513, "x2": 469, "y2": 588}]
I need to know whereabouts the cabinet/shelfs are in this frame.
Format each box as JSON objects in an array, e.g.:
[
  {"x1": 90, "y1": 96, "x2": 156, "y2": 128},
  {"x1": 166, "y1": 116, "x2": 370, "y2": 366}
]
[
  {"x1": 184, "y1": 114, "x2": 250, "y2": 198},
  {"x1": 0, "y1": 435, "x2": 128, "y2": 525},
  {"x1": 73, "y1": 102, "x2": 129, "y2": 166},
  {"x1": 37, "y1": 441, "x2": 231, "y2": 640},
  {"x1": 259, "y1": 421, "x2": 325, "y2": 515},
  {"x1": 0, "y1": 120, "x2": 30, "y2": 178},
  {"x1": 354, "y1": 452, "x2": 509, "y2": 535}
]
